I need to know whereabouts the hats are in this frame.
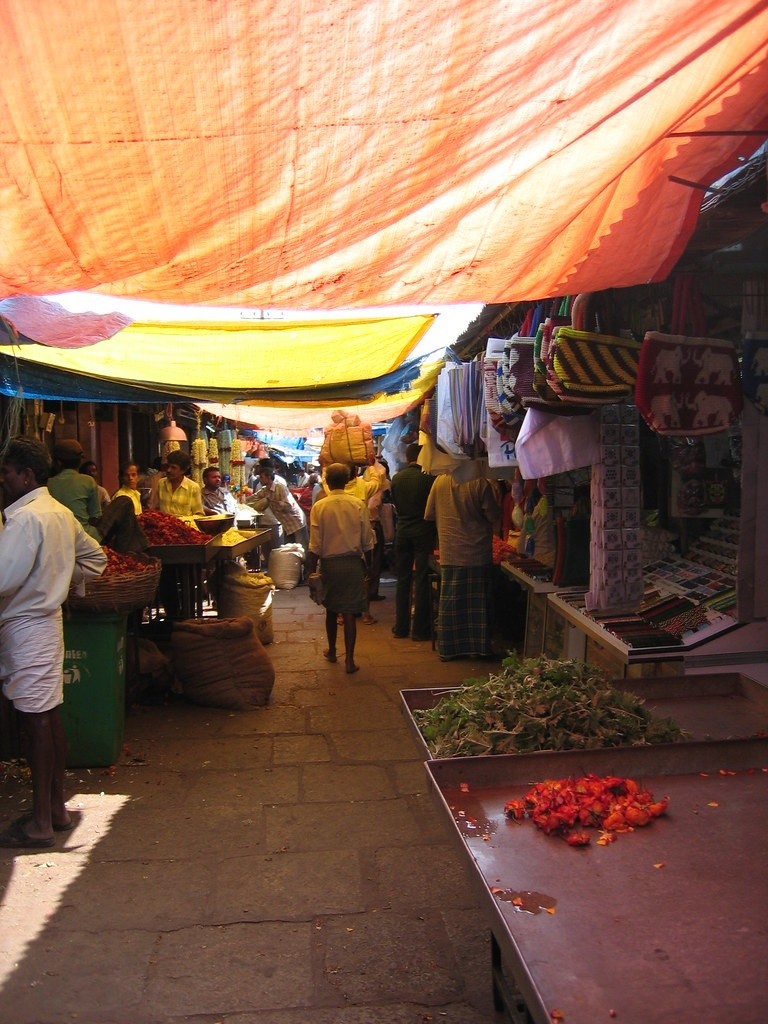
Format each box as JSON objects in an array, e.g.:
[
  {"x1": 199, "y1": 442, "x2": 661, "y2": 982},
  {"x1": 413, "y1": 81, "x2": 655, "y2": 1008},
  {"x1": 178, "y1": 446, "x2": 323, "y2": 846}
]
[{"x1": 57, "y1": 439, "x2": 83, "y2": 459}]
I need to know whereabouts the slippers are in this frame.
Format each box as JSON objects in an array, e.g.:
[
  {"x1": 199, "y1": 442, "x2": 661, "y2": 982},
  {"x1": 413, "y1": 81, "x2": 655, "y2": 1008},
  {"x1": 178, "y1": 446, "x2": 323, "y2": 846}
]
[
  {"x1": 364, "y1": 615, "x2": 376, "y2": 624},
  {"x1": 14, "y1": 813, "x2": 73, "y2": 831},
  {"x1": 0, "y1": 828, "x2": 55, "y2": 847}
]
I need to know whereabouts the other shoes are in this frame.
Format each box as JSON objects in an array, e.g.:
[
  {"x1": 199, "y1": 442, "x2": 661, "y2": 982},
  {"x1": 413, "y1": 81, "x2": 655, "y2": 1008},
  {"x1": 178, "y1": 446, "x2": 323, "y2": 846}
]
[{"x1": 370, "y1": 595, "x2": 386, "y2": 601}]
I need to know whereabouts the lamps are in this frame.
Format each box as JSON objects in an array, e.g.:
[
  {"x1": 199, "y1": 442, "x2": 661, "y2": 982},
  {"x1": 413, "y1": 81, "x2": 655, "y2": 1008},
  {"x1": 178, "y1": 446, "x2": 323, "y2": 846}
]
[{"x1": 159, "y1": 402, "x2": 188, "y2": 443}]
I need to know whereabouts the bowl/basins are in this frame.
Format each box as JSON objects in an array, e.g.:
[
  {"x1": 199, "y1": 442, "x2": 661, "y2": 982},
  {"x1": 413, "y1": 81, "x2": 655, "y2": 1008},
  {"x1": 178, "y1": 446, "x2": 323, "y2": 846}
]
[{"x1": 194, "y1": 513, "x2": 235, "y2": 536}]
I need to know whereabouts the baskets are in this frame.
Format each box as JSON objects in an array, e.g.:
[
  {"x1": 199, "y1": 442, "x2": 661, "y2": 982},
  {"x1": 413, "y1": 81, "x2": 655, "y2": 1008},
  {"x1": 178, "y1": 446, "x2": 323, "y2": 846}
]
[{"x1": 63, "y1": 551, "x2": 162, "y2": 613}]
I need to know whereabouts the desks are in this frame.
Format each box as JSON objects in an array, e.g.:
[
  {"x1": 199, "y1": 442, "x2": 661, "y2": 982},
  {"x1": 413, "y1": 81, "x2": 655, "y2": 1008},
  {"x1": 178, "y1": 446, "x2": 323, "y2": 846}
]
[{"x1": 499, "y1": 561, "x2": 682, "y2": 680}]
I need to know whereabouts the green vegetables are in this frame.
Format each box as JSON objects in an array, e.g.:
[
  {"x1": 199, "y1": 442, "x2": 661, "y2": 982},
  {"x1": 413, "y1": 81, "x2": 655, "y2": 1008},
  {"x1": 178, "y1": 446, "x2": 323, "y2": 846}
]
[{"x1": 411, "y1": 648, "x2": 692, "y2": 756}]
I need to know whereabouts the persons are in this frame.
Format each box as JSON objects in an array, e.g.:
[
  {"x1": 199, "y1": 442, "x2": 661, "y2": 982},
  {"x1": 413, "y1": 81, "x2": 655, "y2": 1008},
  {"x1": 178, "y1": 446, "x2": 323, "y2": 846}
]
[
  {"x1": 311, "y1": 457, "x2": 391, "y2": 673},
  {"x1": 112, "y1": 461, "x2": 142, "y2": 515},
  {"x1": 200, "y1": 466, "x2": 240, "y2": 522},
  {"x1": 243, "y1": 458, "x2": 317, "y2": 584},
  {"x1": 392, "y1": 443, "x2": 435, "y2": 641},
  {"x1": 0, "y1": 436, "x2": 143, "y2": 849},
  {"x1": 424, "y1": 473, "x2": 501, "y2": 660},
  {"x1": 145, "y1": 450, "x2": 204, "y2": 618}
]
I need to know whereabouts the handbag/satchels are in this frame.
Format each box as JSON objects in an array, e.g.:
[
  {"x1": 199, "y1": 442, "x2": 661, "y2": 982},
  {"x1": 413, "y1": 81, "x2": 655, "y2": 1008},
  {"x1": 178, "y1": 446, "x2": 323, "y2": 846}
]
[
  {"x1": 636, "y1": 270, "x2": 742, "y2": 435},
  {"x1": 430, "y1": 290, "x2": 642, "y2": 479}
]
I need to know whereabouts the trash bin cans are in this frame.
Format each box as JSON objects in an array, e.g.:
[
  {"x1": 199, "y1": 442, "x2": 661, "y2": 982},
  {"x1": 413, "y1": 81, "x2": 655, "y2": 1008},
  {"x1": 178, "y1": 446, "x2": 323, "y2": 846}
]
[{"x1": 64, "y1": 608, "x2": 131, "y2": 763}]
